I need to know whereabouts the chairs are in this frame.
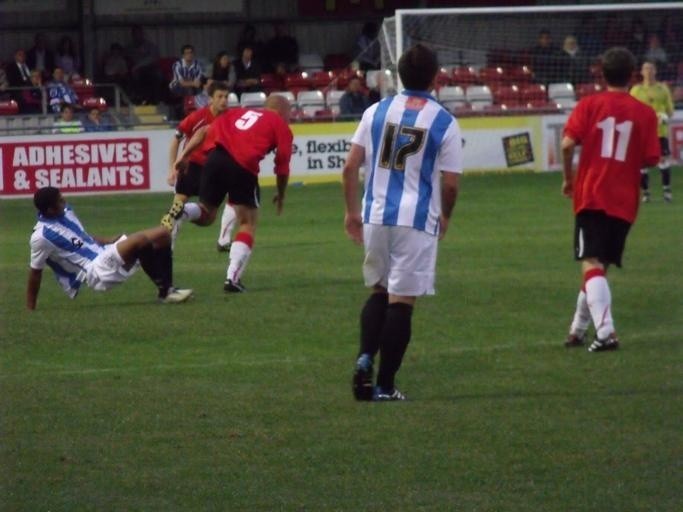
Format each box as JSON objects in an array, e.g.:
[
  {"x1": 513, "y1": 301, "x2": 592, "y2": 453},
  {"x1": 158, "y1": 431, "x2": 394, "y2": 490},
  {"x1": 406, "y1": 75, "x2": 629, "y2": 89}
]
[
  {"x1": 296, "y1": 90, "x2": 325, "y2": 116},
  {"x1": 69, "y1": 79, "x2": 94, "y2": 98},
  {"x1": 481, "y1": 68, "x2": 505, "y2": 88},
  {"x1": 466, "y1": 85, "x2": 493, "y2": 112},
  {"x1": 311, "y1": 72, "x2": 333, "y2": 90},
  {"x1": 285, "y1": 72, "x2": 309, "y2": 91},
  {"x1": 547, "y1": 82, "x2": 577, "y2": 112},
  {"x1": 240, "y1": 92, "x2": 266, "y2": 109},
  {"x1": 494, "y1": 87, "x2": 519, "y2": 109},
  {"x1": 0, "y1": 99, "x2": 19, "y2": 115},
  {"x1": 521, "y1": 84, "x2": 546, "y2": 108},
  {"x1": 453, "y1": 65, "x2": 477, "y2": 88},
  {"x1": 513, "y1": 67, "x2": 535, "y2": 87},
  {"x1": 228, "y1": 93, "x2": 239, "y2": 108},
  {"x1": 82, "y1": 97, "x2": 108, "y2": 112},
  {"x1": 326, "y1": 90, "x2": 346, "y2": 115},
  {"x1": 262, "y1": 74, "x2": 285, "y2": 91},
  {"x1": 438, "y1": 86, "x2": 464, "y2": 113},
  {"x1": 574, "y1": 84, "x2": 596, "y2": 100},
  {"x1": 269, "y1": 91, "x2": 296, "y2": 112}
]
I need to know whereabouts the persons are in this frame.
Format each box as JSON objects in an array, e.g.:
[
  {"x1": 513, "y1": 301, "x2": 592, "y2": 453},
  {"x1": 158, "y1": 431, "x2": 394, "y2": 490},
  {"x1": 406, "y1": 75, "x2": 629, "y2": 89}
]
[
  {"x1": 234, "y1": 46, "x2": 261, "y2": 80},
  {"x1": 195, "y1": 77, "x2": 214, "y2": 108},
  {"x1": 52, "y1": 104, "x2": 85, "y2": 133},
  {"x1": 528, "y1": 16, "x2": 677, "y2": 86},
  {"x1": 167, "y1": 82, "x2": 237, "y2": 253},
  {"x1": 339, "y1": 60, "x2": 396, "y2": 119},
  {"x1": 561, "y1": 48, "x2": 661, "y2": 355},
  {"x1": 340, "y1": 46, "x2": 463, "y2": 405},
  {"x1": 160, "y1": 95, "x2": 294, "y2": 291},
  {"x1": 631, "y1": 57, "x2": 675, "y2": 203},
  {"x1": 26, "y1": 186, "x2": 194, "y2": 312},
  {"x1": 101, "y1": 26, "x2": 165, "y2": 105},
  {"x1": 0, "y1": 32, "x2": 82, "y2": 112},
  {"x1": 83, "y1": 107, "x2": 112, "y2": 131},
  {"x1": 211, "y1": 52, "x2": 236, "y2": 88},
  {"x1": 169, "y1": 44, "x2": 202, "y2": 98}
]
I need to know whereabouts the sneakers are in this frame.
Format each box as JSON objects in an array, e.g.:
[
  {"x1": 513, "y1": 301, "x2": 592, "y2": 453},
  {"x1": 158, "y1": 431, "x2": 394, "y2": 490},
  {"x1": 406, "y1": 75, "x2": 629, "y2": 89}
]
[
  {"x1": 587, "y1": 334, "x2": 619, "y2": 352},
  {"x1": 157, "y1": 288, "x2": 193, "y2": 304},
  {"x1": 160, "y1": 200, "x2": 184, "y2": 232},
  {"x1": 351, "y1": 354, "x2": 374, "y2": 402},
  {"x1": 373, "y1": 384, "x2": 405, "y2": 402},
  {"x1": 563, "y1": 334, "x2": 585, "y2": 348},
  {"x1": 663, "y1": 186, "x2": 672, "y2": 202},
  {"x1": 217, "y1": 241, "x2": 233, "y2": 252},
  {"x1": 642, "y1": 190, "x2": 650, "y2": 203},
  {"x1": 223, "y1": 279, "x2": 246, "y2": 294}
]
